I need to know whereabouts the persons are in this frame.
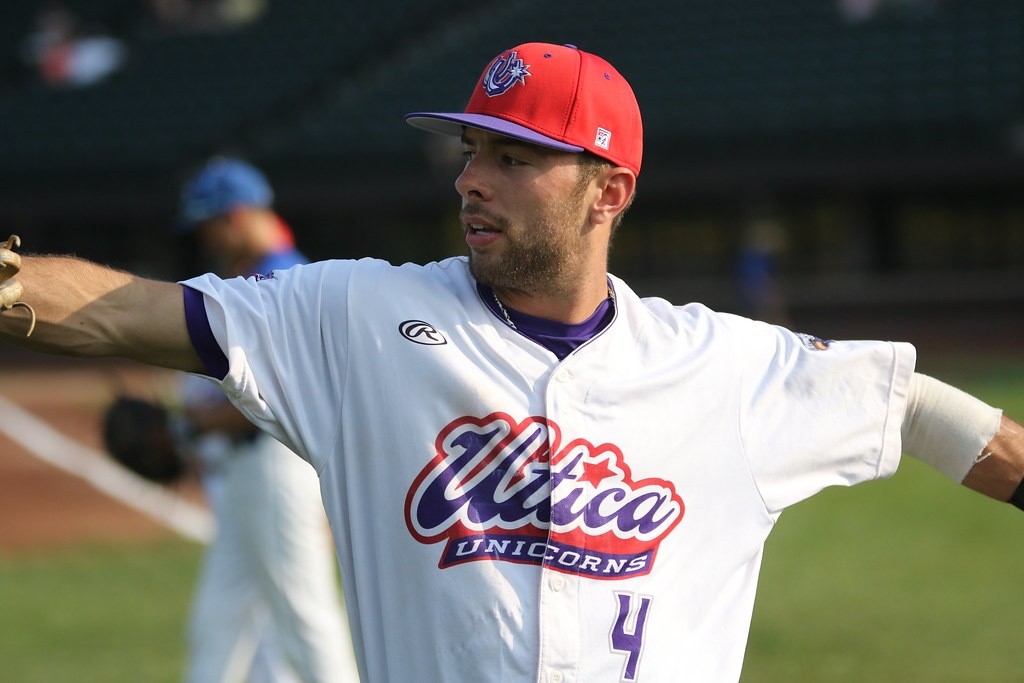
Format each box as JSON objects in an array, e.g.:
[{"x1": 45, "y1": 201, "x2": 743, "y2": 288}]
[
  {"x1": 0, "y1": 42, "x2": 1024, "y2": 683},
  {"x1": 151, "y1": 153, "x2": 357, "y2": 683}
]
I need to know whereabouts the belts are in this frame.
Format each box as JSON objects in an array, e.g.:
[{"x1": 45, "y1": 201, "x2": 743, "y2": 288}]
[{"x1": 232, "y1": 428, "x2": 259, "y2": 447}]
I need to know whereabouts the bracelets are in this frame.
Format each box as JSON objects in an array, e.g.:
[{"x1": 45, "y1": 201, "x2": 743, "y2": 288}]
[{"x1": 162, "y1": 400, "x2": 194, "y2": 442}]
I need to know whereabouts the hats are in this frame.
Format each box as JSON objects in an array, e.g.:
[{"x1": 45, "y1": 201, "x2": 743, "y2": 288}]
[
  {"x1": 183, "y1": 157, "x2": 274, "y2": 221},
  {"x1": 402, "y1": 45, "x2": 644, "y2": 178}
]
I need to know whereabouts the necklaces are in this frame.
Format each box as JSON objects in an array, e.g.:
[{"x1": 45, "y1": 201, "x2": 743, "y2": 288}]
[{"x1": 487, "y1": 284, "x2": 616, "y2": 333}]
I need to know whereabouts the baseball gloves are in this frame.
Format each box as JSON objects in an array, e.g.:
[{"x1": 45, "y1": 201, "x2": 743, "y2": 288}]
[{"x1": 98, "y1": 395, "x2": 197, "y2": 486}]
